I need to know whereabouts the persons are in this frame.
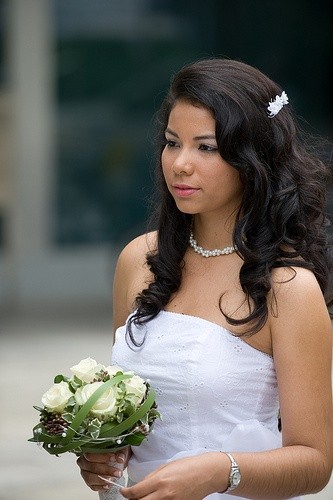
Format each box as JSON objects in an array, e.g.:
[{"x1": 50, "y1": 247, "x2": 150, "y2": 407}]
[{"x1": 76, "y1": 58, "x2": 333, "y2": 500}]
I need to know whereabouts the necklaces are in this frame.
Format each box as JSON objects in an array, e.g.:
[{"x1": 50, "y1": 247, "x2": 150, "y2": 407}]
[{"x1": 188, "y1": 231, "x2": 244, "y2": 257}]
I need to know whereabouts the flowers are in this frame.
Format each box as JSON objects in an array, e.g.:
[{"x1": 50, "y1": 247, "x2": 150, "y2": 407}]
[{"x1": 27, "y1": 356, "x2": 162, "y2": 500}]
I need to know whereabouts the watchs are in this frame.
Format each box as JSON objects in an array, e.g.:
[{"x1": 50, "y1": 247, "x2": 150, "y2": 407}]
[{"x1": 218, "y1": 450, "x2": 242, "y2": 494}]
